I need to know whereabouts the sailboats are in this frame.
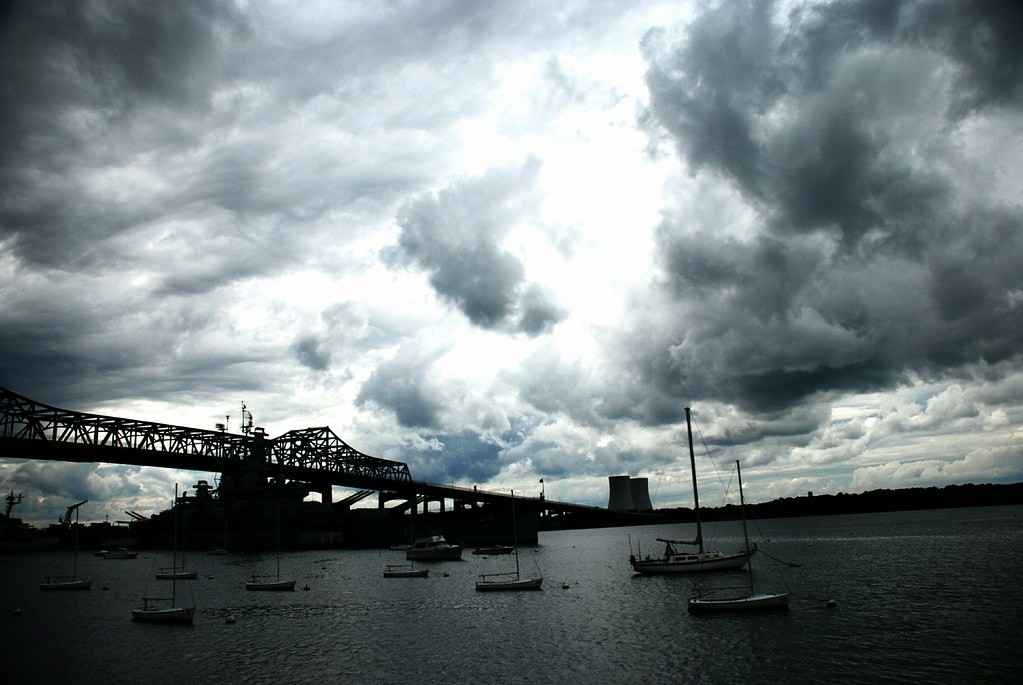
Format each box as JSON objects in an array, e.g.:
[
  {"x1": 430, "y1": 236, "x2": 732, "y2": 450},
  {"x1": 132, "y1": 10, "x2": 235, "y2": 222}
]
[
  {"x1": 475, "y1": 487, "x2": 544, "y2": 593},
  {"x1": 131, "y1": 485, "x2": 197, "y2": 626},
  {"x1": 245, "y1": 499, "x2": 297, "y2": 592},
  {"x1": 207, "y1": 523, "x2": 229, "y2": 556},
  {"x1": 40, "y1": 507, "x2": 91, "y2": 591},
  {"x1": 154, "y1": 492, "x2": 200, "y2": 581},
  {"x1": 686, "y1": 458, "x2": 791, "y2": 618},
  {"x1": 628, "y1": 405, "x2": 760, "y2": 574}
]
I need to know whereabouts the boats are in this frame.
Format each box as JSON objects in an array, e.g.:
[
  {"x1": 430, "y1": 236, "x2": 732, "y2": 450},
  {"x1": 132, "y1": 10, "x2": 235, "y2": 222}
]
[
  {"x1": 405, "y1": 533, "x2": 464, "y2": 561},
  {"x1": 102, "y1": 547, "x2": 139, "y2": 560},
  {"x1": 382, "y1": 568, "x2": 429, "y2": 577},
  {"x1": 118, "y1": 546, "x2": 129, "y2": 551},
  {"x1": 390, "y1": 544, "x2": 411, "y2": 551},
  {"x1": 93, "y1": 549, "x2": 110, "y2": 556},
  {"x1": 473, "y1": 542, "x2": 517, "y2": 556}
]
